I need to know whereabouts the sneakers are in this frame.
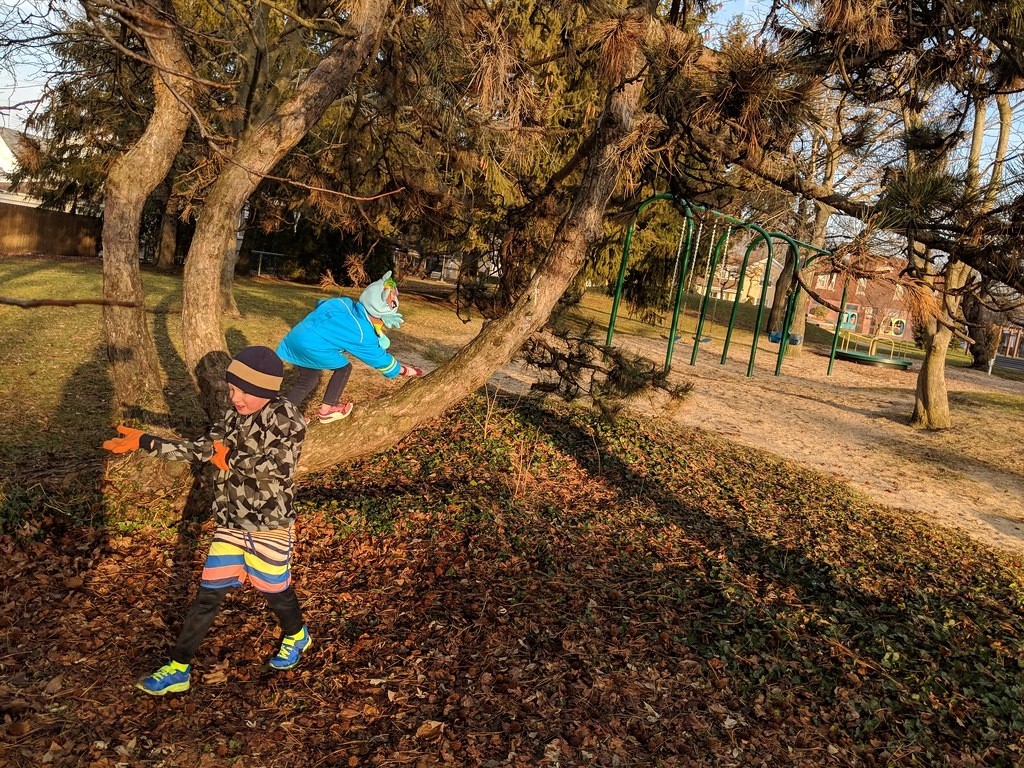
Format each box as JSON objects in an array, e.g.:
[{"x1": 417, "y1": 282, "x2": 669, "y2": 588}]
[
  {"x1": 269, "y1": 625, "x2": 312, "y2": 669},
  {"x1": 304, "y1": 416, "x2": 311, "y2": 425},
  {"x1": 319, "y1": 402, "x2": 353, "y2": 424},
  {"x1": 135, "y1": 660, "x2": 191, "y2": 696}
]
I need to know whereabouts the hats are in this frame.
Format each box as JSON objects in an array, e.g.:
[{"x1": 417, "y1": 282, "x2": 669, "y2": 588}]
[
  {"x1": 226, "y1": 346, "x2": 283, "y2": 399},
  {"x1": 358, "y1": 270, "x2": 404, "y2": 349}
]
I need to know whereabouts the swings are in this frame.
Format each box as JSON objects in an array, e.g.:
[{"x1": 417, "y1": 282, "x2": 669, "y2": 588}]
[
  {"x1": 658, "y1": 210, "x2": 706, "y2": 343},
  {"x1": 690, "y1": 215, "x2": 734, "y2": 344},
  {"x1": 766, "y1": 241, "x2": 819, "y2": 346}
]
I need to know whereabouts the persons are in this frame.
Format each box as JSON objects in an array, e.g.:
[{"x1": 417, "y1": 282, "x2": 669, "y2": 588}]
[
  {"x1": 273, "y1": 270, "x2": 423, "y2": 424},
  {"x1": 103, "y1": 346, "x2": 314, "y2": 698}
]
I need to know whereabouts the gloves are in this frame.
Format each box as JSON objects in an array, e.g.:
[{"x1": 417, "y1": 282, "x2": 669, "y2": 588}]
[
  {"x1": 103, "y1": 426, "x2": 144, "y2": 454},
  {"x1": 210, "y1": 442, "x2": 234, "y2": 470},
  {"x1": 401, "y1": 364, "x2": 424, "y2": 375}
]
